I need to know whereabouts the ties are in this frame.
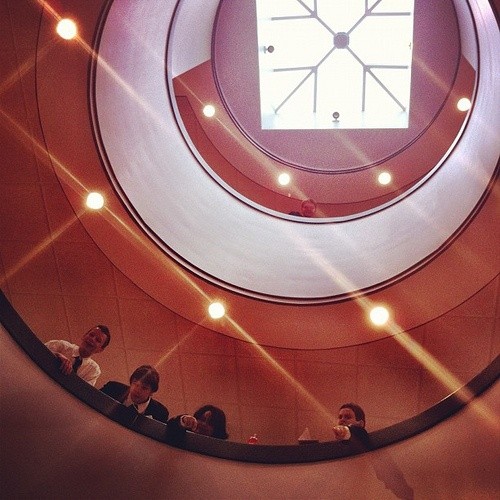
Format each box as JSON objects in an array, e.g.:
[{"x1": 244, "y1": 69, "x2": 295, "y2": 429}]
[{"x1": 73, "y1": 356, "x2": 82, "y2": 373}]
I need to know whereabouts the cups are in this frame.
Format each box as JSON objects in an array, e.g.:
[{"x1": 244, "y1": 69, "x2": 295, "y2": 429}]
[{"x1": 248, "y1": 433, "x2": 258, "y2": 445}]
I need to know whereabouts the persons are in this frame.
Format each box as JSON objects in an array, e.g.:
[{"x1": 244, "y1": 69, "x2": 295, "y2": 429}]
[
  {"x1": 97, "y1": 364, "x2": 169, "y2": 422},
  {"x1": 289, "y1": 198, "x2": 317, "y2": 217},
  {"x1": 332, "y1": 403, "x2": 369, "y2": 441},
  {"x1": 167, "y1": 404, "x2": 230, "y2": 440},
  {"x1": 45, "y1": 324, "x2": 111, "y2": 389}
]
